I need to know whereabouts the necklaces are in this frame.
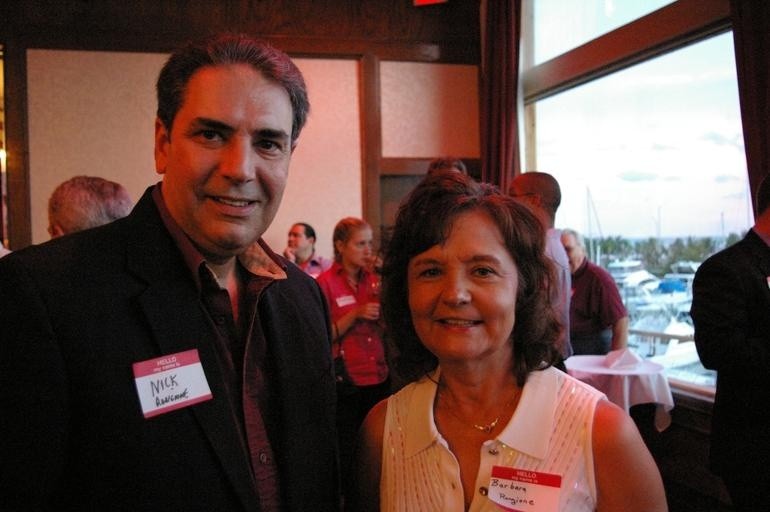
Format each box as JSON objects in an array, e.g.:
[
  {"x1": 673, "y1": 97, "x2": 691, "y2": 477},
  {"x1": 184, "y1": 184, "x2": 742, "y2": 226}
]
[{"x1": 438, "y1": 392, "x2": 518, "y2": 433}]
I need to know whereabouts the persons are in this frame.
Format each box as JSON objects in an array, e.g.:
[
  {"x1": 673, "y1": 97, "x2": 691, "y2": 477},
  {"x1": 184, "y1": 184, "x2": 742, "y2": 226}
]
[
  {"x1": 690, "y1": 170, "x2": 770, "y2": 510},
  {"x1": 316, "y1": 217, "x2": 389, "y2": 463},
  {"x1": 47, "y1": 177, "x2": 132, "y2": 241},
  {"x1": 560, "y1": 230, "x2": 629, "y2": 356},
  {"x1": 282, "y1": 224, "x2": 334, "y2": 280},
  {"x1": 509, "y1": 171, "x2": 572, "y2": 373},
  {"x1": 356, "y1": 171, "x2": 667, "y2": 511},
  {"x1": 0, "y1": 32, "x2": 354, "y2": 508},
  {"x1": 426, "y1": 159, "x2": 469, "y2": 174}
]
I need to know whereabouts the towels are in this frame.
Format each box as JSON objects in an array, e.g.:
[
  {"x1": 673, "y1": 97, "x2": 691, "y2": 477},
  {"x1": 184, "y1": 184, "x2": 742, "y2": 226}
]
[{"x1": 605, "y1": 347, "x2": 644, "y2": 370}]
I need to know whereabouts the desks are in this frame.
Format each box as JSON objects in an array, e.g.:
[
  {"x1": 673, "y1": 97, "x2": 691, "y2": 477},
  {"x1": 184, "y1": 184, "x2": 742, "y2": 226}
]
[{"x1": 562, "y1": 354, "x2": 677, "y2": 432}]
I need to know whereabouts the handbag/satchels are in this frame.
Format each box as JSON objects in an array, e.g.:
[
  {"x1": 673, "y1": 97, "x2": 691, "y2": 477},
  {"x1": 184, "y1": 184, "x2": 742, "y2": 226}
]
[{"x1": 334, "y1": 353, "x2": 357, "y2": 398}]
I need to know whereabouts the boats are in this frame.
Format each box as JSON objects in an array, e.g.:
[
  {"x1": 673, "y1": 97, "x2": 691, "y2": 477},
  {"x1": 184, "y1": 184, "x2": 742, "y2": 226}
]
[{"x1": 603, "y1": 255, "x2": 704, "y2": 367}]
